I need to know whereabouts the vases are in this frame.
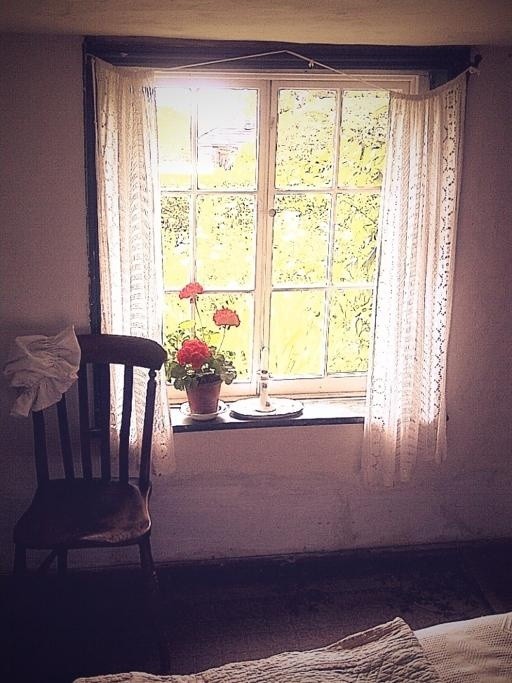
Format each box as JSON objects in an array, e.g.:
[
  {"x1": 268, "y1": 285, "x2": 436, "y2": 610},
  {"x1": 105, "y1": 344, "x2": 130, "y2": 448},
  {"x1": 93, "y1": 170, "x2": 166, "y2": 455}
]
[{"x1": 183, "y1": 377, "x2": 223, "y2": 413}]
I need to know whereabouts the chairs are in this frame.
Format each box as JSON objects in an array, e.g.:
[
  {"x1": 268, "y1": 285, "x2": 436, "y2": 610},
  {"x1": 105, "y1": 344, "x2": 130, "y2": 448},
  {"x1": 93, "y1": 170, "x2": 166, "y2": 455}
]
[{"x1": 12, "y1": 334, "x2": 171, "y2": 675}]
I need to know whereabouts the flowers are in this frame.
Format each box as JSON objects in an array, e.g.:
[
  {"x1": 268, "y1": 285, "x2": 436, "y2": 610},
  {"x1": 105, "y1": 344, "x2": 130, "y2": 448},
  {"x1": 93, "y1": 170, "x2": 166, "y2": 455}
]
[{"x1": 162, "y1": 283, "x2": 240, "y2": 390}]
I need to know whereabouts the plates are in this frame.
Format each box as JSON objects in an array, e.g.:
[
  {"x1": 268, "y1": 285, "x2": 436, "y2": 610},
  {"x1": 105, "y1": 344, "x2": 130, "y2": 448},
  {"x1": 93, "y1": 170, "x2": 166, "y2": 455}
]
[
  {"x1": 228, "y1": 397, "x2": 304, "y2": 419},
  {"x1": 177, "y1": 398, "x2": 229, "y2": 422}
]
